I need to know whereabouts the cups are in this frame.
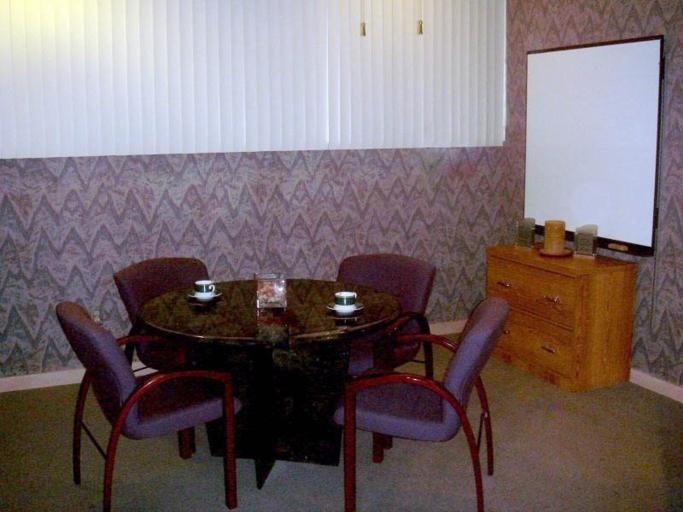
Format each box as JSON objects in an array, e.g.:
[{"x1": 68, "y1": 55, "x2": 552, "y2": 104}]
[
  {"x1": 255, "y1": 273, "x2": 287, "y2": 309},
  {"x1": 544, "y1": 220, "x2": 566, "y2": 253},
  {"x1": 332, "y1": 319, "x2": 355, "y2": 329},
  {"x1": 193, "y1": 279, "x2": 215, "y2": 297},
  {"x1": 333, "y1": 292, "x2": 357, "y2": 311}
]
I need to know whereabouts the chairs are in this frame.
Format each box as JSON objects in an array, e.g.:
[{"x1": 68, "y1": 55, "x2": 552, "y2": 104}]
[
  {"x1": 113, "y1": 258, "x2": 209, "y2": 453},
  {"x1": 56, "y1": 302, "x2": 244, "y2": 512},
  {"x1": 337, "y1": 254, "x2": 435, "y2": 449}
]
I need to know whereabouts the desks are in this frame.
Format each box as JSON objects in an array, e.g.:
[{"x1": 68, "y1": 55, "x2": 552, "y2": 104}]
[
  {"x1": 333, "y1": 297, "x2": 510, "y2": 512},
  {"x1": 139, "y1": 276, "x2": 402, "y2": 491}
]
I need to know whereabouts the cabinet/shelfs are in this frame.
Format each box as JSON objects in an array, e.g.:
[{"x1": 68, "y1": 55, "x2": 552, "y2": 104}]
[{"x1": 485, "y1": 245, "x2": 642, "y2": 395}]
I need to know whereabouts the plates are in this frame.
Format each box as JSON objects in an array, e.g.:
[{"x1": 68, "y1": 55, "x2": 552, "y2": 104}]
[
  {"x1": 325, "y1": 301, "x2": 363, "y2": 315},
  {"x1": 324, "y1": 311, "x2": 361, "y2": 320},
  {"x1": 539, "y1": 248, "x2": 573, "y2": 257},
  {"x1": 185, "y1": 288, "x2": 223, "y2": 302},
  {"x1": 185, "y1": 299, "x2": 222, "y2": 307}
]
